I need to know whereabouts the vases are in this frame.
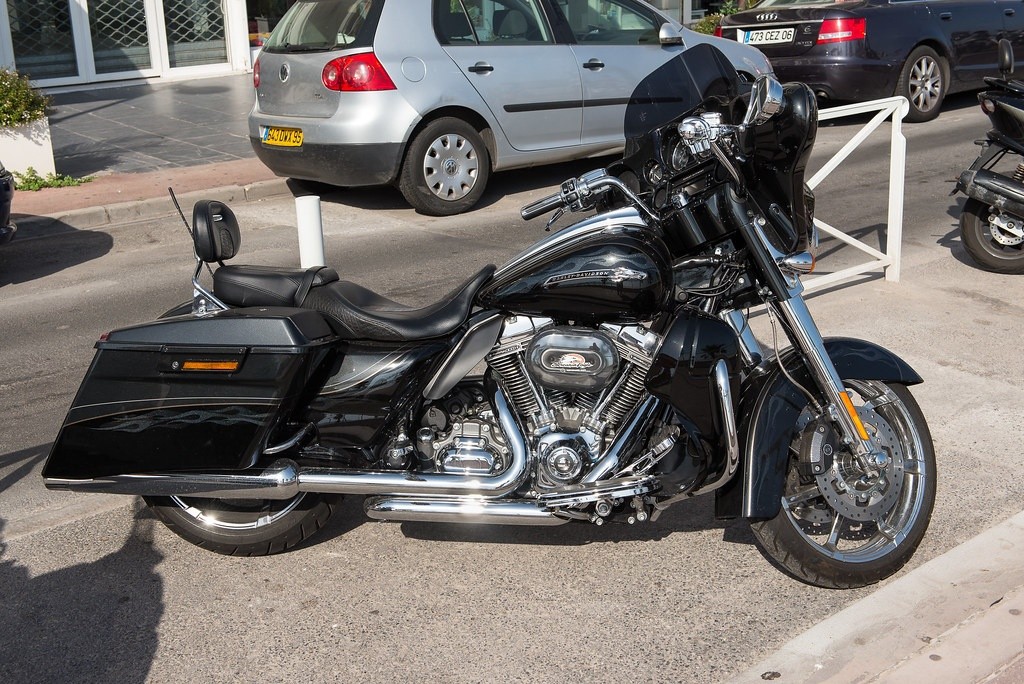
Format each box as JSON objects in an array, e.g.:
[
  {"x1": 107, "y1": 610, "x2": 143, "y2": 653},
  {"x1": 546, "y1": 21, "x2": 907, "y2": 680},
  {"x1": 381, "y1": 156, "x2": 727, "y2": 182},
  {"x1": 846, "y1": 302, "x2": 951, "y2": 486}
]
[{"x1": 0, "y1": 115, "x2": 56, "y2": 185}]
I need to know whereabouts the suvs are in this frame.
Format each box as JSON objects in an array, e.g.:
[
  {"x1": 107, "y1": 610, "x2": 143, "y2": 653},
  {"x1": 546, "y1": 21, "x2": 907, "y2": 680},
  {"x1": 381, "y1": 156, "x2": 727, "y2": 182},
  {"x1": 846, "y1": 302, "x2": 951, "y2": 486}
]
[{"x1": 248, "y1": 0, "x2": 777, "y2": 214}]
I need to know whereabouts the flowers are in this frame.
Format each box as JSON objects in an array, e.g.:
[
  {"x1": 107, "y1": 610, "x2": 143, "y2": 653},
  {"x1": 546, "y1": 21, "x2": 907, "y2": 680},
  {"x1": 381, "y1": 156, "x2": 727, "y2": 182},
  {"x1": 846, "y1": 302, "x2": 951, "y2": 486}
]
[{"x1": 0, "y1": 65, "x2": 50, "y2": 126}]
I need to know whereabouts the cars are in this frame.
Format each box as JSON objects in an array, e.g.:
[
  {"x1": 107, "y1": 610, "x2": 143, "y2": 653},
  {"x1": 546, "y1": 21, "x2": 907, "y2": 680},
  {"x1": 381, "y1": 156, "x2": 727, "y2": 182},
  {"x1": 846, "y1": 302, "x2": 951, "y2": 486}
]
[{"x1": 714, "y1": 0, "x2": 1024, "y2": 123}]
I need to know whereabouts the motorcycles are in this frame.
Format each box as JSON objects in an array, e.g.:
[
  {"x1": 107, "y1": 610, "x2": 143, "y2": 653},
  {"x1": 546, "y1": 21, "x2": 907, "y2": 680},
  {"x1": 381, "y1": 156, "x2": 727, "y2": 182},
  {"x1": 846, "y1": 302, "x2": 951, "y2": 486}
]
[
  {"x1": 943, "y1": 37, "x2": 1024, "y2": 272},
  {"x1": 40, "y1": 42, "x2": 938, "y2": 589}
]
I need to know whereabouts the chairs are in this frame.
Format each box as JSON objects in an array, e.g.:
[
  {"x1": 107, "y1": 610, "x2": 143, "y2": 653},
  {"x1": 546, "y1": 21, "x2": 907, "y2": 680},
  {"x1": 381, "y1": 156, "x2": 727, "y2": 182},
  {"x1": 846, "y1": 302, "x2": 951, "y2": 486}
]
[
  {"x1": 492, "y1": 9, "x2": 528, "y2": 41},
  {"x1": 446, "y1": 13, "x2": 472, "y2": 41}
]
